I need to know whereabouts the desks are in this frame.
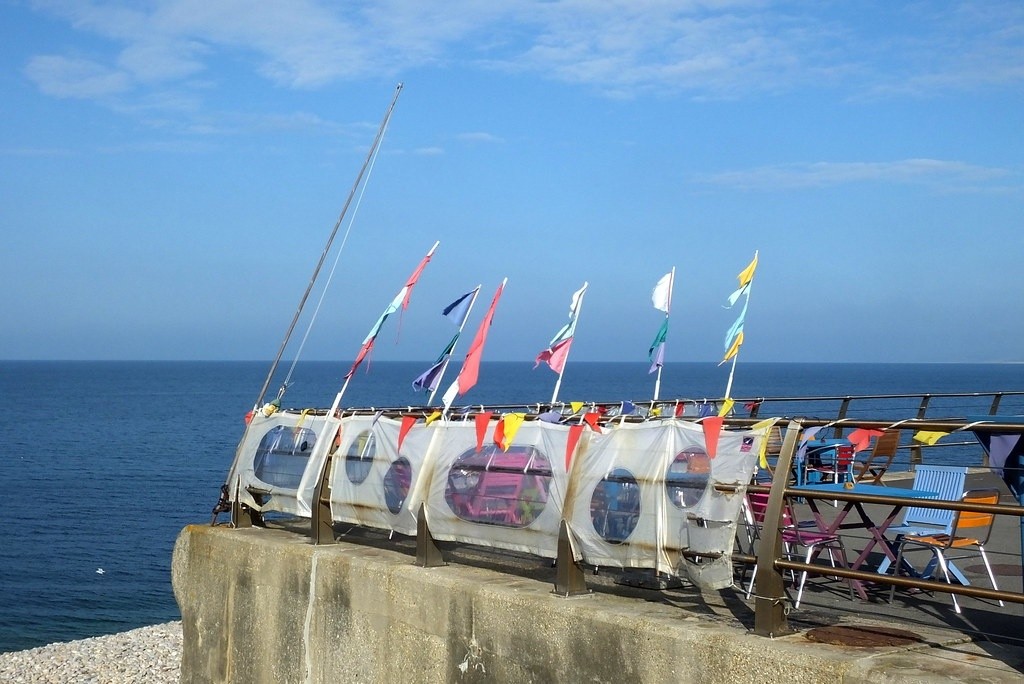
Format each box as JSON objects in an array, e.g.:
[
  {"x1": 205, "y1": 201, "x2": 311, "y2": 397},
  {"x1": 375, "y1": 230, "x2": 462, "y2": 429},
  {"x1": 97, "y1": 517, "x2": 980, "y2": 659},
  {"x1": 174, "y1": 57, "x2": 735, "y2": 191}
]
[
  {"x1": 787, "y1": 483, "x2": 937, "y2": 600},
  {"x1": 773, "y1": 438, "x2": 852, "y2": 485}
]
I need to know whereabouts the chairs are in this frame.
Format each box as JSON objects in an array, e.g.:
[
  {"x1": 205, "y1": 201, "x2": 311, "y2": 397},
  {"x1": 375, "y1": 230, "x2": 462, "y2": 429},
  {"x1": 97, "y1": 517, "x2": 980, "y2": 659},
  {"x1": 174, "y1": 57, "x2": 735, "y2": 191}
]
[
  {"x1": 533, "y1": 459, "x2": 548, "y2": 506},
  {"x1": 764, "y1": 426, "x2": 798, "y2": 482},
  {"x1": 890, "y1": 487, "x2": 1004, "y2": 614},
  {"x1": 803, "y1": 443, "x2": 858, "y2": 507},
  {"x1": 744, "y1": 481, "x2": 855, "y2": 608},
  {"x1": 878, "y1": 465, "x2": 970, "y2": 587},
  {"x1": 457, "y1": 453, "x2": 530, "y2": 523},
  {"x1": 853, "y1": 429, "x2": 902, "y2": 486}
]
